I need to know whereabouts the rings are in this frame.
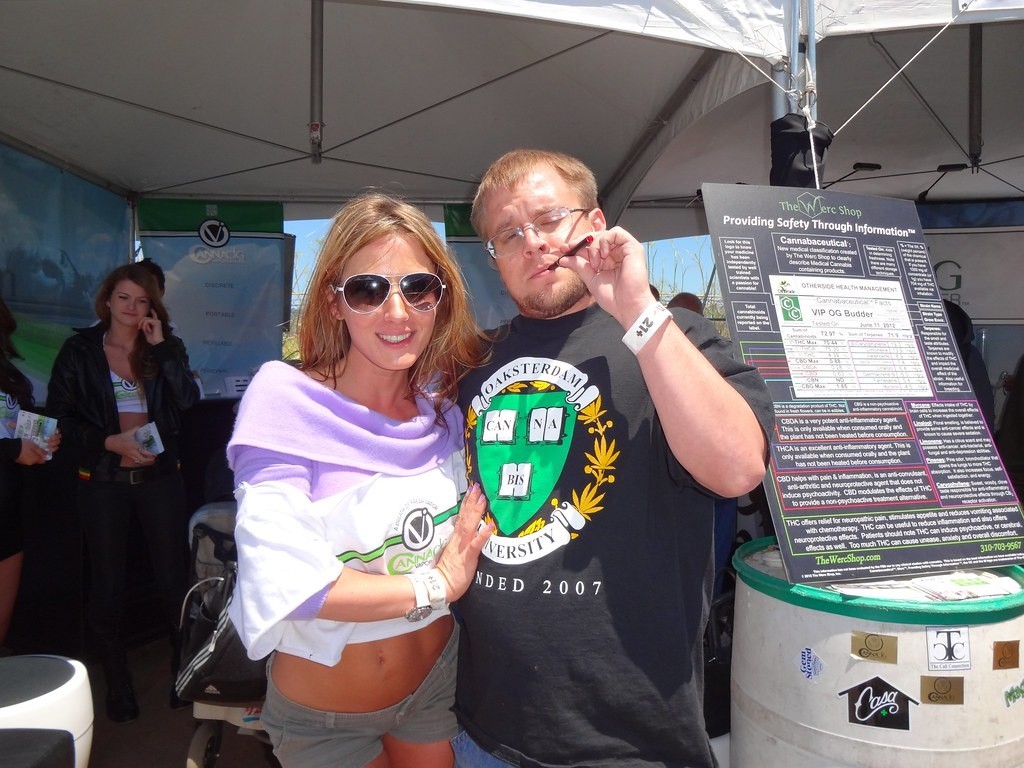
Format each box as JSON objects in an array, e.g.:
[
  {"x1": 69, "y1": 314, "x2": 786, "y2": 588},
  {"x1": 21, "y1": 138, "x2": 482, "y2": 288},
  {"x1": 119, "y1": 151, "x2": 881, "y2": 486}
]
[
  {"x1": 138, "y1": 447, "x2": 142, "y2": 454},
  {"x1": 133, "y1": 459, "x2": 142, "y2": 464}
]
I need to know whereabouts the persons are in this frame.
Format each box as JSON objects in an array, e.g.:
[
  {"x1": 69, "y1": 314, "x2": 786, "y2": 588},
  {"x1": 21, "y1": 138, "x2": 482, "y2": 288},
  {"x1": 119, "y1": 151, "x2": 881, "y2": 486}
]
[
  {"x1": 225, "y1": 189, "x2": 492, "y2": 768},
  {"x1": 436, "y1": 148, "x2": 776, "y2": 768},
  {"x1": 44, "y1": 259, "x2": 205, "y2": 724},
  {"x1": 0, "y1": 300, "x2": 62, "y2": 653}
]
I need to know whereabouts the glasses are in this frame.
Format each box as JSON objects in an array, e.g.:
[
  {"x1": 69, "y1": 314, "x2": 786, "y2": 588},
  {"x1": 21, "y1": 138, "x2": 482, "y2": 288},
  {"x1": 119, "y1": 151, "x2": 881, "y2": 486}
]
[
  {"x1": 485, "y1": 207, "x2": 591, "y2": 259},
  {"x1": 330, "y1": 272, "x2": 446, "y2": 315}
]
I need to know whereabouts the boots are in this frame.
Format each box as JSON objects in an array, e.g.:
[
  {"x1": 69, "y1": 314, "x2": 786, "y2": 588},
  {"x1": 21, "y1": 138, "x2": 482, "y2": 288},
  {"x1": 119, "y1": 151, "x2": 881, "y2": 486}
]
[
  {"x1": 170, "y1": 623, "x2": 193, "y2": 709},
  {"x1": 94, "y1": 619, "x2": 139, "y2": 722}
]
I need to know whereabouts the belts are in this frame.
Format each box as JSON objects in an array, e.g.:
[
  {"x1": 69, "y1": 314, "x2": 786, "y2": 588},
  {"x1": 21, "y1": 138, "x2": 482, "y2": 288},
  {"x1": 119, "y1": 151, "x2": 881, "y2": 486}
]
[{"x1": 107, "y1": 464, "x2": 171, "y2": 485}]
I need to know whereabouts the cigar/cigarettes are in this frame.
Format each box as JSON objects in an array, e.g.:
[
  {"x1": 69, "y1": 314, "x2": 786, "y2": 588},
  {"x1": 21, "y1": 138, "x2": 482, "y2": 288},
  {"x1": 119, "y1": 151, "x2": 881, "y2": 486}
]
[{"x1": 547, "y1": 235, "x2": 595, "y2": 271}]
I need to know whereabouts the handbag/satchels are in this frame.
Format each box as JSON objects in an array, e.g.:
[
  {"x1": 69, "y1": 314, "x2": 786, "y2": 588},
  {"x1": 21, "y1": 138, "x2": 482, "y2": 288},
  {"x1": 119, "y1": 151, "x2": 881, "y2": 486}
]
[{"x1": 175, "y1": 565, "x2": 271, "y2": 702}]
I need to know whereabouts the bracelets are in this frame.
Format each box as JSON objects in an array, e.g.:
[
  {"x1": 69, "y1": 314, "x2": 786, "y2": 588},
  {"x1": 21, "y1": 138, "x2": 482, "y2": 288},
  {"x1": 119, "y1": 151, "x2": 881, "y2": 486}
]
[
  {"x1": 423, "y1": 569, "x2": 446, "y2": 609},
  {"x1": 620, "y1": 299, "x2": 674, "y2": 355}
]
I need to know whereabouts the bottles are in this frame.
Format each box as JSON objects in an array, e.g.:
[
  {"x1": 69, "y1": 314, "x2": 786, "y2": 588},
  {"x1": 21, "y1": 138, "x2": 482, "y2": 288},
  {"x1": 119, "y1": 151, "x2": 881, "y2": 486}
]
[{"x1": 978, "y1": 327, "x2": 992, "y2": 365}]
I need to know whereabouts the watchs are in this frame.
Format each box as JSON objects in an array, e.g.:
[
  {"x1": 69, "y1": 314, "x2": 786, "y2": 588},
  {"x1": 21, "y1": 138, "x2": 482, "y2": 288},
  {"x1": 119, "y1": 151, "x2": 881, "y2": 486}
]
[{"x1": 404, "y1": 573, "x2": 433, "y2": 622}]
